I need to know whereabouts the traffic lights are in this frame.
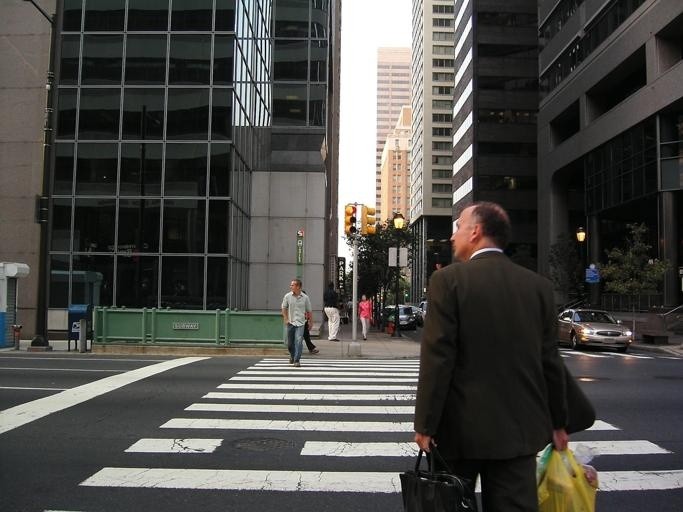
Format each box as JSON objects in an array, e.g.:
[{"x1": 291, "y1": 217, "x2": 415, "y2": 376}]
[
  {"x1": 361, "y1": 206, "x2": 376, "y2": 235},
  {"x1": 345, "y1": 204, "x2": 356, "y2": 235}
]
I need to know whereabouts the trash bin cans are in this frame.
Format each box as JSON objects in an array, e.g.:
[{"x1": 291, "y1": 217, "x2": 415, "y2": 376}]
[
  {"x1": 387, "y1": 315, "x2": 395, "y2": 334},
  {"x1": 67, "y1": 303, "x2": 93, "y2": 350}
]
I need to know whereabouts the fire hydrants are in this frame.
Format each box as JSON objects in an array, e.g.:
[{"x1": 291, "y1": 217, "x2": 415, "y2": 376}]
[{"x1": 386, "y1": 320, "x2": 395, "y2": 334}]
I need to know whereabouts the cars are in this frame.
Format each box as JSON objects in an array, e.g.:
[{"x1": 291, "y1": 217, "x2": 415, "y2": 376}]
[
  {"x1": 558, "y1": 308, "x2": 633, "y2": 351},
  {"x1": 380, "y1": 301, "x2": 428, "y2": 330}
]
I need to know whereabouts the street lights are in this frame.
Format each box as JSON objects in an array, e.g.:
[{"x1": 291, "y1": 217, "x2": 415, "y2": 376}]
[
  {"x1": 577, "y1": 226, "x2": 586, "y2": 309},
  {"x1": 391, "y1": 212, "x2": 405, "y2": 339}
]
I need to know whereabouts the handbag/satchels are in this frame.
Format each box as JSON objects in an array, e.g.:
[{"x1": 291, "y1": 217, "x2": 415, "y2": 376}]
[{"x1": 400, "y1": 445, "x2": 478, "y2": 512}]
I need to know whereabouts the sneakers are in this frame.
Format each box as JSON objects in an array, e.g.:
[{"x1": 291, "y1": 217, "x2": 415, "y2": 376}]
[
  {"x1": 363, "y1": 336, "x2": 367, "y2": 340},
  {"x1": 312, "y1": 348, "x2": 319, "y2": 353},
  {"x1": 330, "y1": 339, "x2": 339, "y2": 341},
  {"x1": 290, "y1": 358, "x2": 300, "y2": 367}
]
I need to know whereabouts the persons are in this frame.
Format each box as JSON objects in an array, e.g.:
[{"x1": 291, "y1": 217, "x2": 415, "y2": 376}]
[
  {"x1": 357, "y1": 295, "x2": 373, "y2": 340},
  {"x1": 281, "y1": 279, "x2": 353, "y2": 367},
  {"x1": 410, "y1": 200, "x2": 596, "y2": 512}
]
